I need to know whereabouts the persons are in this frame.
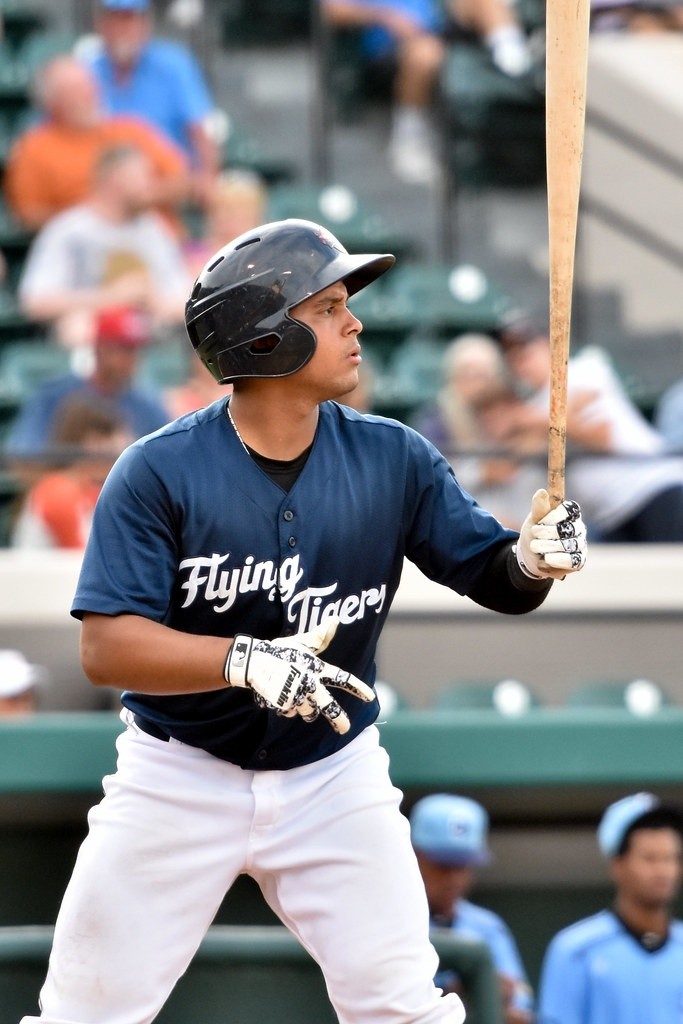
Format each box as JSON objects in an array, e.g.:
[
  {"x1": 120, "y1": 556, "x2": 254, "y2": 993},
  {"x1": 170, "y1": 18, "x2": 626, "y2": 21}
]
[
  {"x1": 407, "y1": 791, "x2": 683, "y2": 1024},
  {"x1": 0, "y1": 647, "x2": 46, "y2": 713},
  {"x1": 0, "y1": 0, "x2": 682, "y2": 551},
  {"x1": 19, "y1": 216, "x2": 588, "y2": 1024}
]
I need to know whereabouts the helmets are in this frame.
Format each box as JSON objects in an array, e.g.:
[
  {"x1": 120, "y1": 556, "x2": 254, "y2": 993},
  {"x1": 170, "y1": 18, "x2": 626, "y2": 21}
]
[{"x1": 184, "y1": 218, "x2": 395, "y2": 385}]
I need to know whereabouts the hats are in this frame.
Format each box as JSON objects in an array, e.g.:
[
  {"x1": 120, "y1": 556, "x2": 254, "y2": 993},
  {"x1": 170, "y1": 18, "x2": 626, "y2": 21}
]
[{"x1": 412, "y1": 794, "x2": 490, "y2": 868}]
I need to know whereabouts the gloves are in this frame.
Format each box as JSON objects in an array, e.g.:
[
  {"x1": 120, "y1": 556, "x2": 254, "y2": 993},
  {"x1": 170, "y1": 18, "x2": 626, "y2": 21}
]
[
  {"x1": 515, "y1": 489, "x2": 587, "y2": 580},
  {"x1": 224, "y1": 614, "x2": 375, "y2": 732}
]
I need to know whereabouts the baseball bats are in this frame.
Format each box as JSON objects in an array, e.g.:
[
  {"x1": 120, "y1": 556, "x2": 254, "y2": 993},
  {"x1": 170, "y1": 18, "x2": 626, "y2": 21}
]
[{"x1": 536, "y1": 0, "x2": 592, "y2": 576}]
